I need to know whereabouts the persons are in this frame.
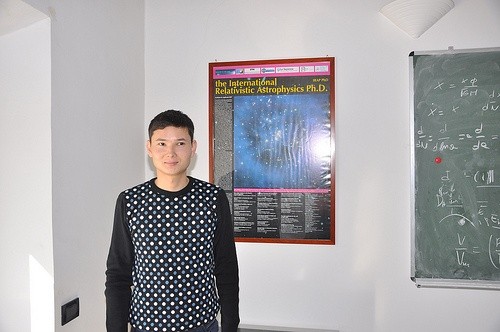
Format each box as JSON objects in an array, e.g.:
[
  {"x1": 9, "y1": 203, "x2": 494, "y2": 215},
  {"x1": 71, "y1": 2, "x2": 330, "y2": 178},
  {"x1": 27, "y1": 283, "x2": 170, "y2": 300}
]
[{"x1": 104, "y1": 110, "x2": 240, "y2": 332}]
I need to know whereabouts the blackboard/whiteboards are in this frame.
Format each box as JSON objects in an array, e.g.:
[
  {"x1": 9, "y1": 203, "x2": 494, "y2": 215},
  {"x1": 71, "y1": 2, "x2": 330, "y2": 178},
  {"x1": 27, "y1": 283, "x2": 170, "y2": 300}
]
[{"x1": 408, "y1": 46, "x2": 500, "y2": 291}]
